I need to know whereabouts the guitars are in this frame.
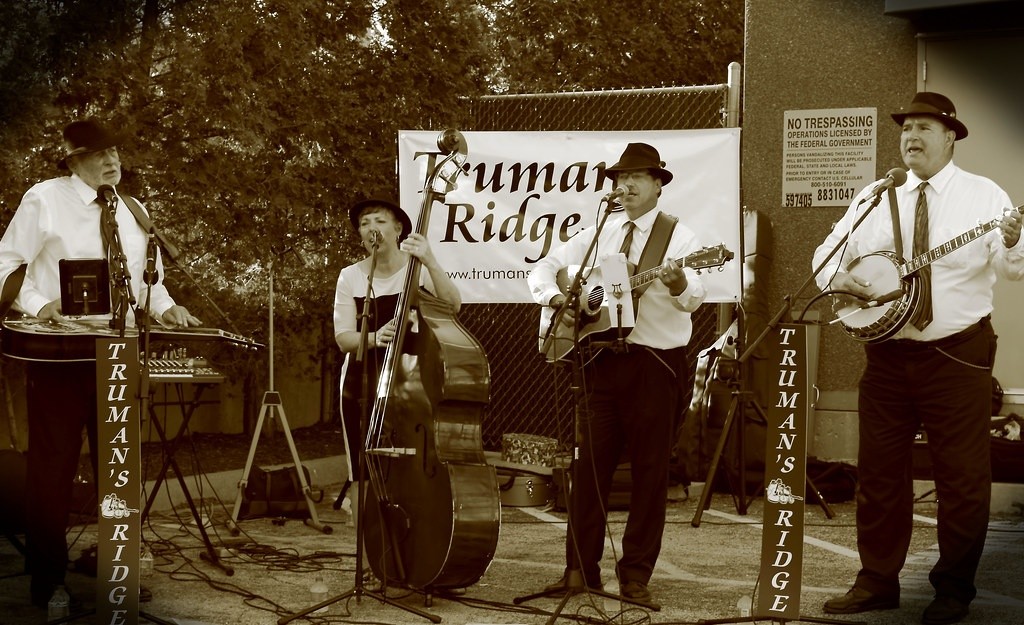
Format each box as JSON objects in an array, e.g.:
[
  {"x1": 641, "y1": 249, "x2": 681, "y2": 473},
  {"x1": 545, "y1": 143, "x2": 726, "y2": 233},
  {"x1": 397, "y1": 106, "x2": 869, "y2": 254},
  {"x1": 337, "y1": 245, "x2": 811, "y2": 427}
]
[
  {"x1": 537, "y1": 241, "x2": 736, "y2": 373},
  {"x1": 829, "y1": 205, "x2": 1023, "y2": 347}
]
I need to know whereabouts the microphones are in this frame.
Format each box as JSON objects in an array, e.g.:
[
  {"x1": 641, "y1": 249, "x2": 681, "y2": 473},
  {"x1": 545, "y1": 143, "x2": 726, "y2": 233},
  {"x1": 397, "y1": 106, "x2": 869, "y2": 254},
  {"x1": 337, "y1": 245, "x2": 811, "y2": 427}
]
[
  {"x1": 601, "y1": 185, "x2": 629, "y2": 201},
  {"x1": 860, "y1": 167, "x2": 907, "y2": 204},
  {"x1": 372, "y1": 229, "x2": 385, "y2": 244},
  {"x1": 97, "y1": 184, "x2": 119, "y2": 202}
]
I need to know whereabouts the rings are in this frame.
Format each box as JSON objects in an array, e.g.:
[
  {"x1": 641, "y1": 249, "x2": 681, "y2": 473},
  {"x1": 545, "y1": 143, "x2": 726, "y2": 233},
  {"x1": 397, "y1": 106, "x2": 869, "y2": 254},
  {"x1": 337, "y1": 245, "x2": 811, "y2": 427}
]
[
  {"x1": 662, "y1": 273, "x2": 667, "y2": 277},
  {"x1": 416, "y1": 247, "x2": 419, "y2": 250}
]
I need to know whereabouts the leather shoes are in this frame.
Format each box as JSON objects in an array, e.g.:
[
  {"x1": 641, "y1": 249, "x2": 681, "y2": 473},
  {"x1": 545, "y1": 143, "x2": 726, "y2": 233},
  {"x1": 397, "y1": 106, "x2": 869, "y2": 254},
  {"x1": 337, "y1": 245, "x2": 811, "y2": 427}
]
[
  {"x1": 919, "y1": 595, "x2": 969, "y2": 625},
  {"x1": 822, "y1": 585, "x2": 900, "y2": 613}
]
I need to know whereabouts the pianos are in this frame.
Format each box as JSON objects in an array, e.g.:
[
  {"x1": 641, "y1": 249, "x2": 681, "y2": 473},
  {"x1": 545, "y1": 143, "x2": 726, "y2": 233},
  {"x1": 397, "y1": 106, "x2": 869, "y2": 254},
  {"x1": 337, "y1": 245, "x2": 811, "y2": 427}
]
[{"x1": 0, "y1": 313, "x2": 240, "y2": 577}]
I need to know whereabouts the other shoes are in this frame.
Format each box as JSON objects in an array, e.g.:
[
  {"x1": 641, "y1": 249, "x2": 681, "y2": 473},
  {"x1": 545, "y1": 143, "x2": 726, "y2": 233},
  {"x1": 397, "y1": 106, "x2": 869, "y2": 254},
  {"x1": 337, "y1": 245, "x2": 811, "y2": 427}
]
[
  {"x1": 31, "y1": 582, "x2": 73, "y2": 605},
  {"x1": 622, "y1": 580, "x2": 651, "y2": 602},
  {"x1": 544, "y1": 564, "x2": 601, "y2": 598}
]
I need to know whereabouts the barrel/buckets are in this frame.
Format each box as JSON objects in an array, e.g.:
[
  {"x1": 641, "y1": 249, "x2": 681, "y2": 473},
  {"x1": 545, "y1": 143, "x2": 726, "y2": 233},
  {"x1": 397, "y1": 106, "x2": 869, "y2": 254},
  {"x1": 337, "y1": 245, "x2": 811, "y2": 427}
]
[{"x1": 552, "y1": 468, "x2": 572, "y2": 508}]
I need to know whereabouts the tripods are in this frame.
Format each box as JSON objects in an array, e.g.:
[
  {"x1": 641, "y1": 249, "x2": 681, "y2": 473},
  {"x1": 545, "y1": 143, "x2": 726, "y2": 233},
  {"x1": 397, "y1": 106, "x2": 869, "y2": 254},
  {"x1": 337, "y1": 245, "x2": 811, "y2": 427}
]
[
  {"x1": 691, "y1": 304, "x2": 835, "y2": 526},
  {"x1": 514, "y1": 196, "x2": 660, "y2": 625},
  {"x1": 43, "y1": 198, "x2": 242, "y2": 625},
  {"x1": 277, "y1": 243, "x2": 442, "y2": 625}
]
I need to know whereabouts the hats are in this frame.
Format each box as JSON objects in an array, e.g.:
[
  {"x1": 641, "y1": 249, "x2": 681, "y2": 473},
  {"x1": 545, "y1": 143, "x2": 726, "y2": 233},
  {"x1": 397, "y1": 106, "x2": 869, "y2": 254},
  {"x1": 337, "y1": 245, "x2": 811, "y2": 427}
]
[
  {"x1": 891, "y1": 92, "x2": 969, "y2": 141},
  {"x1": 349, "y1": 200, "x2": 413, "y2": 244},
  {"x1": 604, "y1": 143, "x2": 674, "y2": 187},
  {"x1": 57, "y1": 121, "x2": 128, "y2": 170}
]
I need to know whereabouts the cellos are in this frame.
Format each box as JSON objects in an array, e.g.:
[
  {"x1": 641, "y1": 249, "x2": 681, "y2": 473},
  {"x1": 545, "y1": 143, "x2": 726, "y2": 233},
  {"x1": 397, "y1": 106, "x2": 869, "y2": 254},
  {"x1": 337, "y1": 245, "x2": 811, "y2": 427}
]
[{"x1": 360, "y1": 125, "x2": 503, "y2": 596}]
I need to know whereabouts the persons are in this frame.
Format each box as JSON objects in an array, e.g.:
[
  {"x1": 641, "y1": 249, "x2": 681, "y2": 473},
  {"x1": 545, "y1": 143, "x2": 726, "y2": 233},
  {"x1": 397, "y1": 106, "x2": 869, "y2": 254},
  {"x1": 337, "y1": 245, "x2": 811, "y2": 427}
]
[
  {"x1": 334, "y1": 192, "x2": 462, "y2": 582},
  {"x1": 527, "y1": 142, "x2": 706, "y2": 602},
  {"x1": 0, "y1": 120, "x2": 203, "y2": 601},
  {"x1": 812, "y1": 92, "x2": 1024, "y2": 615}
]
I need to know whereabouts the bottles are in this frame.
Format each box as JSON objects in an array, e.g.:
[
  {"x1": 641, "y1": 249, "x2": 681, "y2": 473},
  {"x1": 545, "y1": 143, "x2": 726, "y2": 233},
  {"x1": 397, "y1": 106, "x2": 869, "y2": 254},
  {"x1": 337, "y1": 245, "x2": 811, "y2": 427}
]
[
  {"x1": 311, "y1": 577, "x2": 328, "y2": 613},
  {"x1": 736, "y1": 592, "x2": 753, "y2": 625},
  {"x1": 139, "y1": 546, "x2": 153, "y2": 579},
  {"x1": 48, "y1": 592, "x2": 66, "y2": 625},
  {"x1": 55, "y1": 586, "x2": 70, "y2": 625}
]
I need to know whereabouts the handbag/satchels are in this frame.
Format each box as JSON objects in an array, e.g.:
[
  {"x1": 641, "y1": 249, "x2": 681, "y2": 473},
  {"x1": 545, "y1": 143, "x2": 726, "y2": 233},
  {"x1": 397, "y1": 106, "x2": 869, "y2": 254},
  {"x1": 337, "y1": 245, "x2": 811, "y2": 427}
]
[{"x1": 242, "y1": 464, "x2": 312, "y2": 519}]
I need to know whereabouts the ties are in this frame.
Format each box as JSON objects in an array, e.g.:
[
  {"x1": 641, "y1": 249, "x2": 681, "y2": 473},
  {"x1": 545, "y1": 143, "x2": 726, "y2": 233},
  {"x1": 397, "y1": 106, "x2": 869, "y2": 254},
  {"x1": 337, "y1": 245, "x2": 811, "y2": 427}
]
[
  {"x1": 94, "y1": 198, "x2": 128, "y2": 318},
  {"x1": 909, "y1": 182, "x2": 934, "y2": 331},
  {"x1": 619, "y1": 223, "x2": 636, "y2": 259}
]
